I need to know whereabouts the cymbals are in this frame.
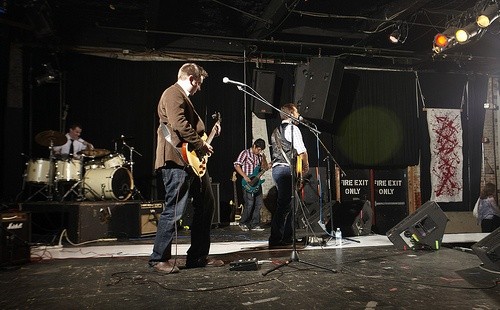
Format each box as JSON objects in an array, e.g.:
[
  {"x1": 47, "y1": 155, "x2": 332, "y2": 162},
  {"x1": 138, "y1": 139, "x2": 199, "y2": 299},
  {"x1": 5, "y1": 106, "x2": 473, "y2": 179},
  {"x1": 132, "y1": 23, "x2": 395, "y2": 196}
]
[
  {"x1": 103, "y1": 153, "x2": 128, "y2": 168},
  {"x1": 36, "y1": 130, "x2": 68, "y2": 148},
  {"x1": 77, "y1": 149, "x2": 111, "y2": 156},
  {"x1": 112, "y1": 137, "x2": 135, "y2": 142}
]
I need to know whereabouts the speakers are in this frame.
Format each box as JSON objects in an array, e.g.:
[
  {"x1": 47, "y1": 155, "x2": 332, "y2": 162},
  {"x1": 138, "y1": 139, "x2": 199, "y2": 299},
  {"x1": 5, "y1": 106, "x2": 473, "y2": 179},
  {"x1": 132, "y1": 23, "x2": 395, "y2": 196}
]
[
  {"x1": 298, "y1": 54, "x2": 346, "y2": 128},
  {"x1": 253, "y1": 70, "x2": 279, "y2": 119},
  {"x1": 387, "y1": 201, "x2": 449, "y2": 251},
  {"x1": 471, "y1": 228, "x2": 500, "y2": 271},
  {"x1": 326, "y1": 201, "x2": 373, "y2": 236},
  {"x1": 122, "y1": 202, "x2": 165, "y2": 237},
  {"x1": 181, "y1": 183, "x2": 220, "y2": 227},
  {"x1": 0, "y1": 209, "x2": 32, "y2": 266}
]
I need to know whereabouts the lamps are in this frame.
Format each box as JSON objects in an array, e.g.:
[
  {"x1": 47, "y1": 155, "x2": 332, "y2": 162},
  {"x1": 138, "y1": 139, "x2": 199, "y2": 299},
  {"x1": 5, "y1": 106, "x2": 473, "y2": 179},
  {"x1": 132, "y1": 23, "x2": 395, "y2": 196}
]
[
  {"x1": 477, "y1": 3, "x2": 500, "y2": 27},
  {"x1": 454, "y1": 22, "x2": 481, "y2": 42},
  {"x1": 435, "y1": 28, "x2": 458, "y2": 48},
  {"x1": 389, "y1": 25, "x2": 402, "y2": 44}
]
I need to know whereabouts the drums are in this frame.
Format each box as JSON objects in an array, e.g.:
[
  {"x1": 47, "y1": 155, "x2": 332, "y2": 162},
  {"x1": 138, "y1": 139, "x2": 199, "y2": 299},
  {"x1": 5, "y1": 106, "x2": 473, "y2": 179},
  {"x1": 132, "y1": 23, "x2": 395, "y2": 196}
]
[
  {"x1": 84, "y1": 160, "x2": 105, "y2": 171},
  {"x1": 24, "y1": 158, "x2": 55, "y2": 188},
  {"x1": 82, "y1": 166, "x2": 134, "y2": 201},
  {"x1": 55, "y1": 156, "x2": 82, "y2": 184}
]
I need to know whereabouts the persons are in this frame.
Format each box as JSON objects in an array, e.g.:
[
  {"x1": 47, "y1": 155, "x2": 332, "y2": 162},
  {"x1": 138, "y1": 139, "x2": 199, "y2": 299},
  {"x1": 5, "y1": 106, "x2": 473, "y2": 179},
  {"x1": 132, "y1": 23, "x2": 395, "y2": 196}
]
[
  {"x1": 472, "y1": 183, "x2": 500, "y2": 233},
  {"x1": 268, "y1": 104, "x2": 309, "y2": 249},
  {"x1": 233, "y1": 138, "x2": 268, "y2": 232},
  {"x1": 148, "y1": 63, "x2": 224, "y2": 274},
  {"x1": 52, "y1": 123, "x2": 94, "y2": 156}
]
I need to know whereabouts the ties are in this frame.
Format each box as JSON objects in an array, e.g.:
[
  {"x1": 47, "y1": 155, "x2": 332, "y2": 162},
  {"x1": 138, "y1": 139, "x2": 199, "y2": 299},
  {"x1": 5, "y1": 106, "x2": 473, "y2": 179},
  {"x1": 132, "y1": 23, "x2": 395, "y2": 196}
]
[{"x1": 69, "y1": 138, "x2": 75, "y2": 154}]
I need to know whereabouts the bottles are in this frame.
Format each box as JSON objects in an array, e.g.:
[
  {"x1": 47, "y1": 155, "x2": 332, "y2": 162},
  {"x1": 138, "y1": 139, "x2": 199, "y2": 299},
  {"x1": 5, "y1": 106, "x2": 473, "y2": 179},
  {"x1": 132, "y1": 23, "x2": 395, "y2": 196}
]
[{"x1": 335, "y1": 228, "x2": 342, "y2": 246}]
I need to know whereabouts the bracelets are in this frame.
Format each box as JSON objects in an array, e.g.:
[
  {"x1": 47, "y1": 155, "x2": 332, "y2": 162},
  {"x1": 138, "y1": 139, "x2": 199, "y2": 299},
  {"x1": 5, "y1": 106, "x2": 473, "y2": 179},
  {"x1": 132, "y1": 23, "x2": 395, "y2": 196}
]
[{"x1": 303, "y1": 164, "x2": 309, "y2": 168}]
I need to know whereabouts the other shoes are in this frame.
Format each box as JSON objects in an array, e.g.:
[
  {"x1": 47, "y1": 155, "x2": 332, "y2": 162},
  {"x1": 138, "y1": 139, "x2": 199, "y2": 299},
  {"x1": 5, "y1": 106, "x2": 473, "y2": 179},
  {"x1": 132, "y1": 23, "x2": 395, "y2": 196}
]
[
  {"x1": 186, "y1": 255, "x2": 224, "y2": 267},
  {"x1": 250, "y1": 225, "x2": 265, "y2": 231},
  {"x1": 268, "y1": 240, "x2": 293, "y2": 248},
  {"x1": 238, "y1": 224, "x2": 249, "y2": 231},
  {"x1": 148, "y1": 260, "x2": 179, "y2": 273}
]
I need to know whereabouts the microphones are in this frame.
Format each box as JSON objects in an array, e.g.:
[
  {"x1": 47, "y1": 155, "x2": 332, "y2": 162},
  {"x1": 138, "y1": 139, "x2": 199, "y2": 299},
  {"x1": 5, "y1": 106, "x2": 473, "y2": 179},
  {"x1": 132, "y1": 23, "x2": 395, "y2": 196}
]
[
  {"x1": 298, "y1": 116, "x2": 315, "y2": 125},
  {"x1": 223, "y1": 77, "x2": 248, "y2": 86}
]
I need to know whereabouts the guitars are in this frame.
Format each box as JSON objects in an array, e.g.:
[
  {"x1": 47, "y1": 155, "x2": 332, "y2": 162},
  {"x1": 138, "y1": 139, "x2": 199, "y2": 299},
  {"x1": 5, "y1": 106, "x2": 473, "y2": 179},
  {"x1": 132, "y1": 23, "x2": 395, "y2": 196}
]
[
  {"x1": 297, "y1": 155, "x2": 303, "y2": 189},
  {"x1": 241, "y1": 160, "x2": 275, "y2": 194},
  {"x1": 182, "y1": 112, "x2": 222, "y2": 178},
  {"x1": 229, "y1": 172, "x2": 243, "y2": 223}
]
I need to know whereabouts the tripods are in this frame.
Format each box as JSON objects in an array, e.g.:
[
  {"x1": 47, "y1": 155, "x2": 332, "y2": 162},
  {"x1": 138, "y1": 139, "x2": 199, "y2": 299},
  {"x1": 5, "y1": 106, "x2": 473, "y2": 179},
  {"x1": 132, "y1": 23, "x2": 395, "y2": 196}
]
[
  {"x1": 238, "y1": 86, "x2": 337, "y2": 276},
  {"x1": 312, "y1": 125, "x2": 361, "y2": 244}
]
